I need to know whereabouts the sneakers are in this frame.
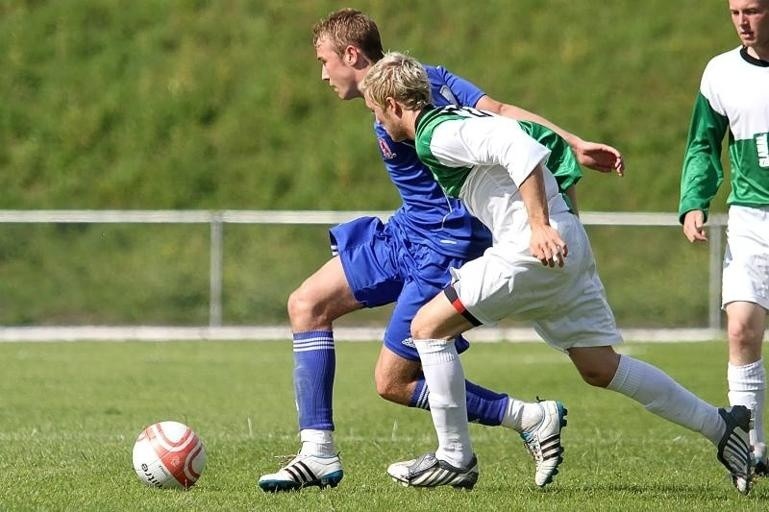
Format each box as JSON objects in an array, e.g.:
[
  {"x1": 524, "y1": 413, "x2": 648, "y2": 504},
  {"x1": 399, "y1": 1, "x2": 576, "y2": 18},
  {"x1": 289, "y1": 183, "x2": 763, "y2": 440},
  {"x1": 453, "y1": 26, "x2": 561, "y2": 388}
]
[
  {"x1": 715, "y1": 401, "x2": 758, "y2": 495},
  {"x1": 256, "y1": 451, "x2": 347, "y2": 494},
  {"x1": 517, "y1": 392, "x2": 570, "y2": 487},
  {"x1": 385, "y1": 451, "x2": 482, "y2": 492}
]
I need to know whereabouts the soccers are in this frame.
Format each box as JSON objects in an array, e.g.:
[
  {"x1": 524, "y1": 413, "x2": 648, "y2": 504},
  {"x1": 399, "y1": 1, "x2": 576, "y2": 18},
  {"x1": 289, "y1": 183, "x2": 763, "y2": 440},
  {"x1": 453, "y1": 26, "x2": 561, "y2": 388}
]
[{"x1": 133, "y1": 421, "x2": 205, "y2": 490}]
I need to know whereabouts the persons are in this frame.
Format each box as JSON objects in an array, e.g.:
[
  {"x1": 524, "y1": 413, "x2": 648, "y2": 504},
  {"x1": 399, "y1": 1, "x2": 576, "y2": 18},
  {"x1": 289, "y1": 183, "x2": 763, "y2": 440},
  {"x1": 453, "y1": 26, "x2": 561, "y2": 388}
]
[
  {"x1": 360, "y1": 49, "x2": 755, "y2": 497},
  {"x1": 252, "y1": 6, "x2": 625, "y2": 487},
  {"x1": 676, "y1": 0, "x2": 768, "y2": 476}
]
